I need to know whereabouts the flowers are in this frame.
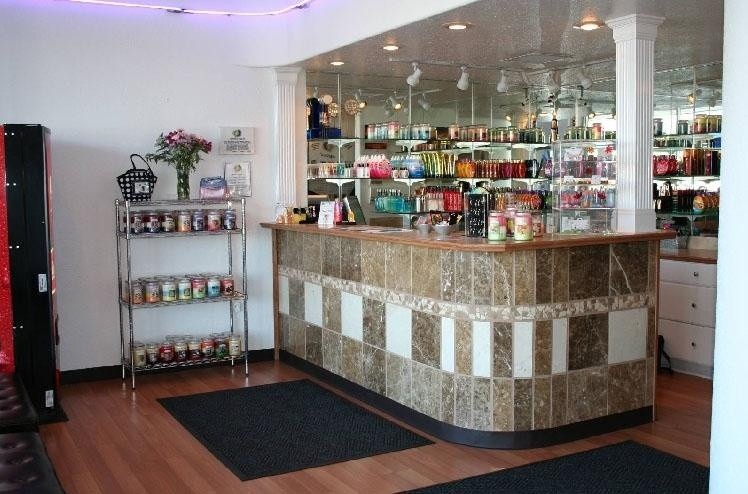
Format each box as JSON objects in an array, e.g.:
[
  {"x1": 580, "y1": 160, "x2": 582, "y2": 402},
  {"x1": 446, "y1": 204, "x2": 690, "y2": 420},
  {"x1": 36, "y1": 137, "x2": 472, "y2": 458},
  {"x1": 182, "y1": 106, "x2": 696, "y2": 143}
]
[{"x1": 146, "y1": 125, "x2": 214, "y2": 190}]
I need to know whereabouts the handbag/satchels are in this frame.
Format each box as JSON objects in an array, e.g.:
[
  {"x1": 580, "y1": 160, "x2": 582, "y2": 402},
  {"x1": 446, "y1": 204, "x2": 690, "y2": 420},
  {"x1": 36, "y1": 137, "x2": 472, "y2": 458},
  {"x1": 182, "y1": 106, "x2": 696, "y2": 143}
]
[
  {"x1": 116, "y1": 169, "x2": 158, "y2": 202},
  {"x1": 199, "y1": 177, "x2": 228, "y2": 201}
]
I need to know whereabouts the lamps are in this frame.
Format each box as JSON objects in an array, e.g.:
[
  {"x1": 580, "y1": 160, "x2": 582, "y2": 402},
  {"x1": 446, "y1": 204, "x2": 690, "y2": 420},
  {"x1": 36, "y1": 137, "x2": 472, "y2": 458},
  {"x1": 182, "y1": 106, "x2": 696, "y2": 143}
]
[{"x1": 386, "y1": 53, "x2": 595, "y2": 92}]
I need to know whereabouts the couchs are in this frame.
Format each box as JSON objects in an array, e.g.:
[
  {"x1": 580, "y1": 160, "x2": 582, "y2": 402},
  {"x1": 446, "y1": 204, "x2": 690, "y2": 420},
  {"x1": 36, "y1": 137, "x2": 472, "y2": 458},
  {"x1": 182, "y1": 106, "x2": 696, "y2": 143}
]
[{"x1": 1, "y1": 377, "x2": 66, "y2": 494}]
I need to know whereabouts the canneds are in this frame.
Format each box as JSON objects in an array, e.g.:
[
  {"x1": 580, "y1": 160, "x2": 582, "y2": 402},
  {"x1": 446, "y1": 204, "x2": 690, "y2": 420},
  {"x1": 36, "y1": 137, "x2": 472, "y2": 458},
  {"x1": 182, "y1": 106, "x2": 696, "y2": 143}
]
[
  {"x1": 488, "y1": 205, "x2": 533, "y2": 242},
  {"x1": 122, "y1": 209, "x2": 241, "y2": 367},
  {"x1": 365, "y1": 113, "x2": 722, "y2": 142}
]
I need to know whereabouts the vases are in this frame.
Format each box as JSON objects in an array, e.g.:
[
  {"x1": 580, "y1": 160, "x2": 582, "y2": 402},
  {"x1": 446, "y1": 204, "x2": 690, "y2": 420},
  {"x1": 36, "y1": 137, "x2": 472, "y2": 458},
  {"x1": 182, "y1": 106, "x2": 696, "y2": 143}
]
[{"x1": 176, "y1": 163, "x2": 191, "y2": 201}]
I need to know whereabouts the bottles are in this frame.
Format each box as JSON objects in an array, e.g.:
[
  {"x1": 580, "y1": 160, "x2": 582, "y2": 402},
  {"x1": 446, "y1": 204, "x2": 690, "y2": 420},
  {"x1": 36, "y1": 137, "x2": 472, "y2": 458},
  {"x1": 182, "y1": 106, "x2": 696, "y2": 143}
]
[
  {"x1": 551, "y1": 115, "x2": 558, "y2": 140},
  {"x1": 335, "y1": 197, "x2": 342, "y2": 224},
  {"x1": 653, "y1": 153, "x2": 677, "y2": 176},
  {"x1": 682, "y1": 148, "x2": 720, "y2": 176},
  {"x1": 677, "y1": 119, "x2": 689, "y2": 135},
  {"x1": 692, "y1": 190, "x2": 719, "y2": 214},
  {"x1": 652, "y1": 118, "x2": 662, "y2": 135},
  {"x1": 375, "y1": 184, "x2": 552, "y2": 213},
  {"x1": 693, "y1": 113, "x2": 721, "y2": 133},
  {"x1": 344, "y1": 151, "x2": 528, "y2": 178},
  {"x1": 290, "y1": 204, "x2": 317, "y2": 223}
]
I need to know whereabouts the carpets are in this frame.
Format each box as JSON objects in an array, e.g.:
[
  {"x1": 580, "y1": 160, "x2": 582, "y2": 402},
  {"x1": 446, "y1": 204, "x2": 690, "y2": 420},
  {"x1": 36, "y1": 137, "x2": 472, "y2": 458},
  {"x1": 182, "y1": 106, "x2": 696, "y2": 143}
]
[
  {"x1": 157, "y1": 378, "x2": 437, "y2": 480},
  {"x1": 393, "y1": 438, "x2": 710, "y2": 492}
]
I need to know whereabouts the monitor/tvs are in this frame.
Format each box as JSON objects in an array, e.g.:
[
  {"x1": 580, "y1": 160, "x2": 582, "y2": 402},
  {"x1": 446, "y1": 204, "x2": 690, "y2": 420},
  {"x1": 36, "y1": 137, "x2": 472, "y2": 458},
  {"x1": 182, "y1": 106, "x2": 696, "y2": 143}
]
[
  {"x1": 308, "y1": 195, "x2": 330, "y2": 206},
  {"x1": 343, "y1": 196, "x2": 366, "y2": 225}
]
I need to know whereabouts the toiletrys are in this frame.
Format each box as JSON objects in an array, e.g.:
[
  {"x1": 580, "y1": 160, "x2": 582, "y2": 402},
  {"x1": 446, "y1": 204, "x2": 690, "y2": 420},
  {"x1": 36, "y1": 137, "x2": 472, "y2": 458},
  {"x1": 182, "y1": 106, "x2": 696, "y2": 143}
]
[
  {"x1": 307, "y1": 159, "x2": 345, "y2": 178},
  {"x1": 344, "y1": 163, "x2": 371, "y2": 178}
]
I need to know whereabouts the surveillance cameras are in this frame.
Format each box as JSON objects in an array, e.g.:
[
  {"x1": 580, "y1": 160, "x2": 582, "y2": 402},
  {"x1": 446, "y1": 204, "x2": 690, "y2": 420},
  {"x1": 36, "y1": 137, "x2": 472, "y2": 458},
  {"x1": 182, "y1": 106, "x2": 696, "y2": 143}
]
[
  {"x1": 548, "y1": 96, "x2": 556, "y2": 103},
  {"x1": 577, "y1": 97, "x2": 586, "y2": 105}
]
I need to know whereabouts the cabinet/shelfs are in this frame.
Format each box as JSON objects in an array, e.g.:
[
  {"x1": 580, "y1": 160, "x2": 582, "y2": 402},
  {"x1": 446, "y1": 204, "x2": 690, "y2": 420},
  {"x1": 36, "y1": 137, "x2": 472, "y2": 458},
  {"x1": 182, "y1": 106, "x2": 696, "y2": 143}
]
[
  {"x1": 115, "y1": 195, "x2": 251, "y2": 388},
  {"x1": 658, "y1": 248, "x2": 718, "y2": 382},
  {"x1": 311, "y1": 125, "x2": 720, "y2": 218}
]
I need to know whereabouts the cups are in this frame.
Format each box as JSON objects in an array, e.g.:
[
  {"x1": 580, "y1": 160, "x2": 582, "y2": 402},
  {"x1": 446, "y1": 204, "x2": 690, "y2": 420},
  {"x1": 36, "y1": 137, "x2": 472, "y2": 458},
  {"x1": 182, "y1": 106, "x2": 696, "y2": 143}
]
[
  {"x1": 414, "y1": 223, "x2": 432, "y2": 233},
  {"x1": 435, "y1": 224, "x2": 451, "y2": 234}
]
